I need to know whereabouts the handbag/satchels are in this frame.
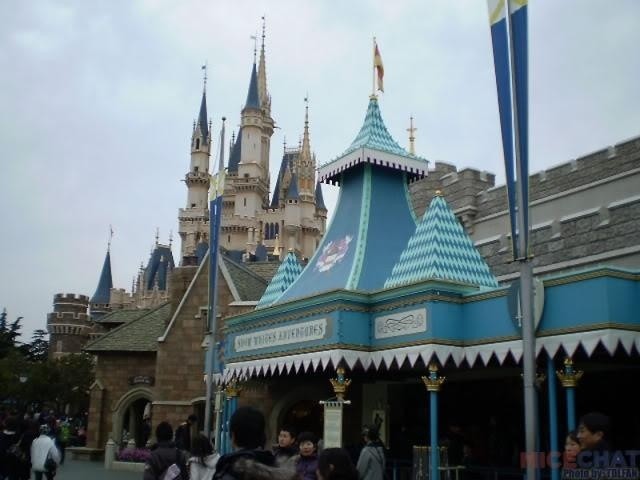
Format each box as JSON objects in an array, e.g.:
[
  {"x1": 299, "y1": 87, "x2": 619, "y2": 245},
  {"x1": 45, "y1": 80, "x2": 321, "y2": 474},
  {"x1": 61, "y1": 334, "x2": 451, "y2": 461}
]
[{"x1": 44, "y1": 459, "x2": 56, "y2": 472}]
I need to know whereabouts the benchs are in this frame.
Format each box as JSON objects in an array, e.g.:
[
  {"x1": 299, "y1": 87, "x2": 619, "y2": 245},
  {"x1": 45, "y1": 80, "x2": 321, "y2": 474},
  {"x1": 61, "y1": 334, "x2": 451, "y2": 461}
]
[{"x1": 64, "y1": 447, "x2": 105, "y2": 460}]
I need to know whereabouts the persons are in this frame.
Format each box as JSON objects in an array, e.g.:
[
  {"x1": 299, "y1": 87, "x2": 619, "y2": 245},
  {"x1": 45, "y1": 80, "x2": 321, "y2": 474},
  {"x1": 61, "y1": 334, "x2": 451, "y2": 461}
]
[{"x1": 2, "y1": 407, "x2": 638, "y2": 480}]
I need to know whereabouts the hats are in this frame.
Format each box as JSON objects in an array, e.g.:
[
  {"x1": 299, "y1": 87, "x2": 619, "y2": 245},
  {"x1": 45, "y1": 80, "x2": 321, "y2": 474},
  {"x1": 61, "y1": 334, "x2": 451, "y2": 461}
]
[{"x1": 38, "y1": 424, "x2": 51, "y2": 434}]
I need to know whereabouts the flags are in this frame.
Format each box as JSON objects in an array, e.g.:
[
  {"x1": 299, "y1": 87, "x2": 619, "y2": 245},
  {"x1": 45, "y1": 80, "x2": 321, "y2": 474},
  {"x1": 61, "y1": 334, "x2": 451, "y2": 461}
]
[{"x1": 375, "y1": 45, "x2": 384, "y2": 92}]
